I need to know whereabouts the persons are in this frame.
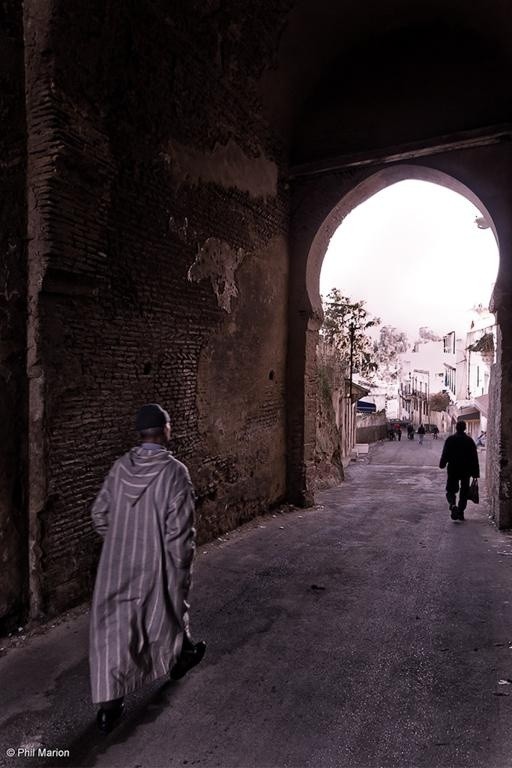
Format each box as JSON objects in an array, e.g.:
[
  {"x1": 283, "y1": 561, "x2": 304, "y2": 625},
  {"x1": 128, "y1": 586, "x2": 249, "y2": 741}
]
[
  {"x1": 386, "y1": 421, "x2": 415, "y2": 442},
  {"x1": 84, "y1": 402, "x2": 207, "y2": 733},
  {"x1": 437, "y1": 420, "x2": 479, "y2": 521},
  {"x1": 416, "y1": 424, "x2": 425, "y2": 445},
  {"x1": 431, "y1": 425, "x2": 439, "y2": 440}
]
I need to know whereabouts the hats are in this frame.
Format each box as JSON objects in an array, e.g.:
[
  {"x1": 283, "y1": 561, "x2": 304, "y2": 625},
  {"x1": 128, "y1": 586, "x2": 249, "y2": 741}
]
[
  {"x1": 456, "y1": 421, "x2": 466, "y2": 431},
  {"x1": 137, "y1": 404, "x2": 170, "y2": 430}
]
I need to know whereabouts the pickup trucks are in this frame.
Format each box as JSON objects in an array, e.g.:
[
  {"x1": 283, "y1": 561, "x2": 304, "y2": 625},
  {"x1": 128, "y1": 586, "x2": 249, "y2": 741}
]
[{"x1": 388, "y1": 418, "x2": 409, "y2": 428}]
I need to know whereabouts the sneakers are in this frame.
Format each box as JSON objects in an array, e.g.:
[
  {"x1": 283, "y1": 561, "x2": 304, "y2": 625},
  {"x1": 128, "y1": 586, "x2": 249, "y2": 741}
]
[{"x1": 451, "y1": 506, "x2": 464, "y2": 520}]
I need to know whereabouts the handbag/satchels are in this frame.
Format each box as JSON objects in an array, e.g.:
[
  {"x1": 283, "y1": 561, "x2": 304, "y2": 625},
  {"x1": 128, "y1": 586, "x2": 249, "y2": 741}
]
[{"x1": 469, "y1": 478, "x2": 479, "y2": 504}]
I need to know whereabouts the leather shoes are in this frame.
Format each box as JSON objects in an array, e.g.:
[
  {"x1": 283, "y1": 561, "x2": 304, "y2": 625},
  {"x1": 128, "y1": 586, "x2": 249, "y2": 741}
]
[
  {"x1": 97, "y1": 700, "x2": 127, "y2": 734},
  {"x1": 173, "y1": 641, "x2": 206, "y2": 680}
]
